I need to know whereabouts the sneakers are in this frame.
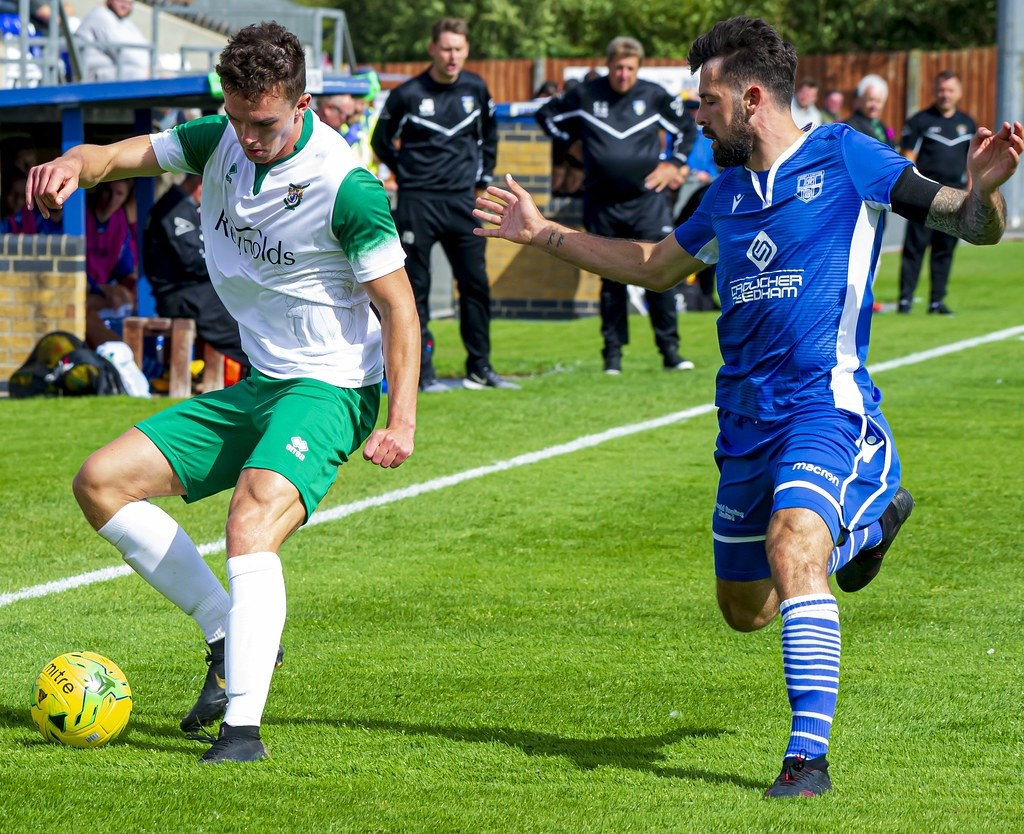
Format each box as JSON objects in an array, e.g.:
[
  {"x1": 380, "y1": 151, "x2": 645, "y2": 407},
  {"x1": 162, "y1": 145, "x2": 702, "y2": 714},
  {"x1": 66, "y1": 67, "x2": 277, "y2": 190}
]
[
  {"x1": 836, "y1": 487, "x2": 914, "y2": 593},
  {"x1": 179, "y1": 638, "x2": 284, "y2": 733},
  {"x1": 462, "y1": 364, "x2": 521, "y2": 391},
  {"x1": 419, "y1": 367, "x2": 452, "y2": 392},
  {"x1": 764, "y1": 748, "x2": 832, "y2": 798},
  {"x1": 184, "y1": 714, "x2": 265, "y2": 764}
]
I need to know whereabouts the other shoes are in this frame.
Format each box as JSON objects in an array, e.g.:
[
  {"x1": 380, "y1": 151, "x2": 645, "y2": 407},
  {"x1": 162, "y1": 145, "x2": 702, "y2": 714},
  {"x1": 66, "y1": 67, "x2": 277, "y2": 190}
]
[
  {"x1": 602, "y1": 349, "x2": 622, "y2": 376},
  {"x1": 928, "y1": 302, "x2": 951, "y2": 314},
  {"x1": 663, "y1": 345, "x2": 695, "y2": 371},
  {"x1": 898, "y1": 299, "x2": 912, "y2": 314}
]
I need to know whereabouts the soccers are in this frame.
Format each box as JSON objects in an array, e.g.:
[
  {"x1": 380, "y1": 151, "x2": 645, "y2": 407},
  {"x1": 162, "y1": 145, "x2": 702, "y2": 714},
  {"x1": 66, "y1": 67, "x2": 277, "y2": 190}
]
[
  {"x1": 30, "y1": 650, "x2": 132, "y2": 749},
  {"x1": 10, "y1": 334, "x2": 99, "y2": 393}
]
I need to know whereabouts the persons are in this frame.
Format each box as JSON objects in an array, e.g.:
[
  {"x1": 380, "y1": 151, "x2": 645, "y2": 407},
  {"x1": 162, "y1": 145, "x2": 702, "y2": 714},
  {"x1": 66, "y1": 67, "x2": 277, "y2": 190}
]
[
  {"x1": 27, "y1": 21, "x2": 420, "y2": 761},
  {"x1": 471, "y1": 17, "x2": 1023, "y2": 800},
  {"x1": 529, "y1": 34, "x2": 977, "y2": 374},
  {"x1": 370, "y1": 16, "x2": 523, "y2": 393},
  {"x1": 0, "y1": 1, "x2": 380, "y2": 397}
]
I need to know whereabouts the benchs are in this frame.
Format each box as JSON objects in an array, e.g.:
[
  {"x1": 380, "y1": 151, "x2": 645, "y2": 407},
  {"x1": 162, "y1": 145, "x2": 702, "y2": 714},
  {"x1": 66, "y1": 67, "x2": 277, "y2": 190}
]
[{"x1": 118, "y1": 317, "x2": 198, "y2": 398}]
